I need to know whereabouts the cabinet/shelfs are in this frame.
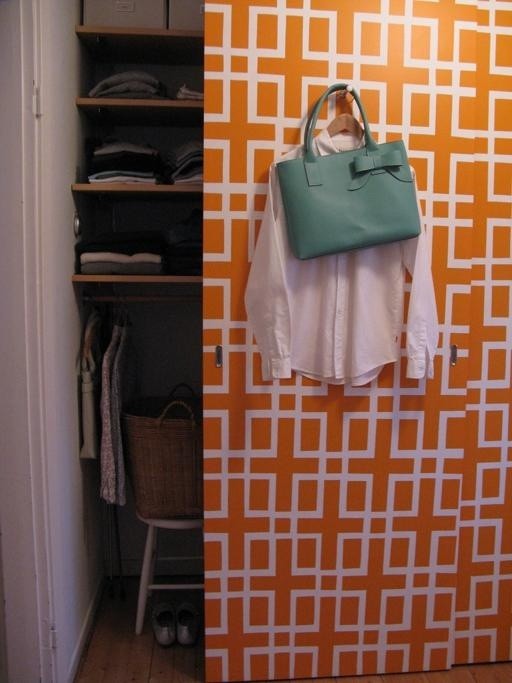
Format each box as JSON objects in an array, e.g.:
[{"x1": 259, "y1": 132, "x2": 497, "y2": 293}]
[{"x1": 75, "y1": 27, "x2": 201, "y2": 284}]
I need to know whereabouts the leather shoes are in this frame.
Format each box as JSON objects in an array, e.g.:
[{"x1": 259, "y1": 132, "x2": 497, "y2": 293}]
[
  {"x1": 175, "y1": 602, "x2": 200, "y2": 649},
  {"x1": 151, "y1": 601, "x2": 177, "y2": 649}
]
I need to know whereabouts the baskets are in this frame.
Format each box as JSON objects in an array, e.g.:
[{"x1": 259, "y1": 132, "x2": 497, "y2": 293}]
[{"x1": 121, "y1": 382, "x2": 204, "y2": 520}]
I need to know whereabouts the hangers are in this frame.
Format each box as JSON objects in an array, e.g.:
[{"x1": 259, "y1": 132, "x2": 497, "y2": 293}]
[
  {"x1": 79, "y1": 295, "x2": 131, "y2": 354},
  {"x1": 325, "y1": 88, "x2": 365, "y2": 140}
]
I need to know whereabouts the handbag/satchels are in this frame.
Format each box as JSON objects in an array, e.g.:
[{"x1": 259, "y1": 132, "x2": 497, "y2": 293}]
[
  {"x1": 79, "y1": 314, "x2": 100, "y2": 461},
  {"x1": 276, "y1": 82, "x2": 422, "y2": 260}
]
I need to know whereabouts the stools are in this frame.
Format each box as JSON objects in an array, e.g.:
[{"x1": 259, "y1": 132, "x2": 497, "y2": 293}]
[{"x1": 135, "y1": 508, "x2": 204, "y2": 638}]
